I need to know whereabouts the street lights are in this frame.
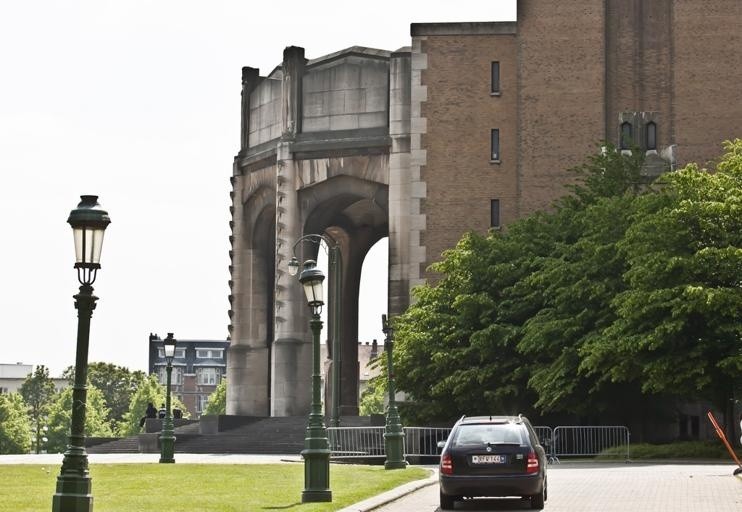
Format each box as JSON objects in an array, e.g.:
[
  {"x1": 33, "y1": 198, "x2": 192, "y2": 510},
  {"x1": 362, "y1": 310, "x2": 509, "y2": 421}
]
[
  {"x1": 51, "y1": 194, "x2": 111, "y2": 512},
  {"x1": 381, "y1": 314, "x2": 406, "y2": 469},
  {"x1": 288, "y1": 234, "x2": 342, "y2": 451},
  {"x1": 158, "y1": 333, "x2": 177, "y2": 463},
  {"x1": 299, "y1": 259, "x2": 333, "y2": 501}
]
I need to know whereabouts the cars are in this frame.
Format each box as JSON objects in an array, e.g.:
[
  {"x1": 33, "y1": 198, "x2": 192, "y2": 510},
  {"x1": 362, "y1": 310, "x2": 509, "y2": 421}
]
[{"x1": 436, "y1": 413, "x2": 550, "y2": 509}]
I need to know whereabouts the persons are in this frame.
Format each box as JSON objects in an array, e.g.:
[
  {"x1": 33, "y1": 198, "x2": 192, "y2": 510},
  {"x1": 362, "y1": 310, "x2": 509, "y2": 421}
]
[
  {"x1": 140, "y1": 403, "x2": 158, "y2": 426},
  {"x1": 173, "y1": 405, "x2": 184, "y2": 419},
  {"x1": 159, "y1": 404, "x2": 167, "y2": 419}
]
